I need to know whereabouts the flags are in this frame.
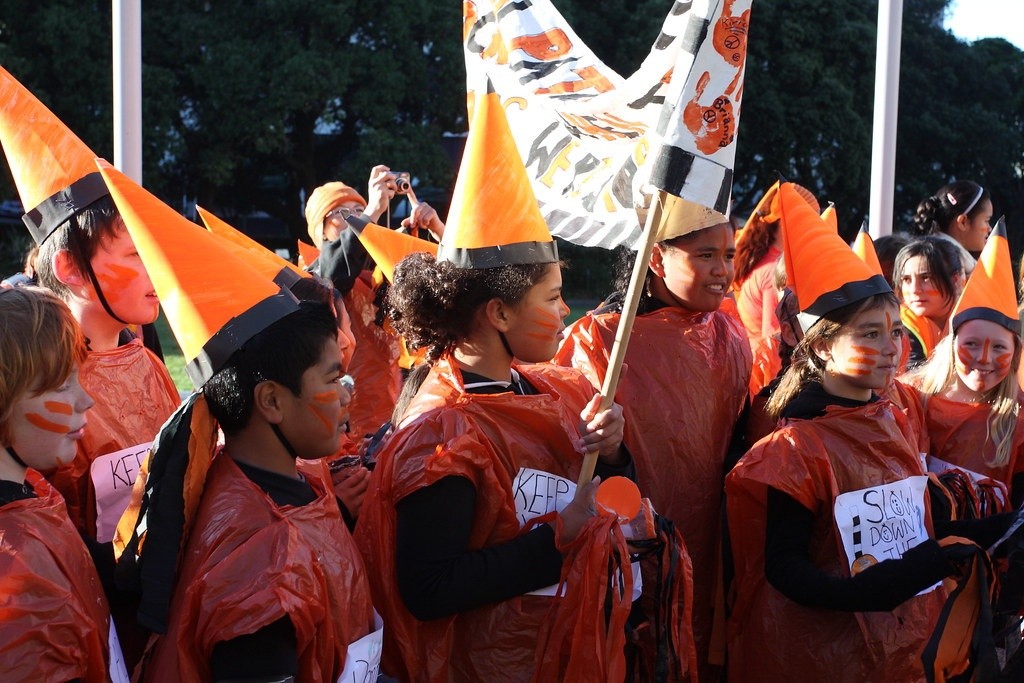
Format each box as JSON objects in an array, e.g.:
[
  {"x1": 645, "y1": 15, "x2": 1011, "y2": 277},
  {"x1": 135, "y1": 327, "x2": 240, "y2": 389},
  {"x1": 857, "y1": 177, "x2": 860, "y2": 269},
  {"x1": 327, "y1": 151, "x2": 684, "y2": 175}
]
[{"x1": 462, "y1": 1, "x2": 749, "y2": 249}]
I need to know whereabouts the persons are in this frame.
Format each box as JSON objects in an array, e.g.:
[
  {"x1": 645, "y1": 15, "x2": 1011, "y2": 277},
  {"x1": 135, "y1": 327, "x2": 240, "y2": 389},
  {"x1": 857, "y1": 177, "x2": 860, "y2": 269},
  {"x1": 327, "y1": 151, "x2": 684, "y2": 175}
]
[
  {"x1": 547, "y1": 191, "x2": 753, "y2": 682},
  {"x1": 0, "y1": 285, "x2": 114, "y2": 683},
  {"x1": 99, "y1": 160, "x2": 375, "y2": 683},
  {"x1": 0, "y1": 71, "x2": 1024, "y2": 683}
]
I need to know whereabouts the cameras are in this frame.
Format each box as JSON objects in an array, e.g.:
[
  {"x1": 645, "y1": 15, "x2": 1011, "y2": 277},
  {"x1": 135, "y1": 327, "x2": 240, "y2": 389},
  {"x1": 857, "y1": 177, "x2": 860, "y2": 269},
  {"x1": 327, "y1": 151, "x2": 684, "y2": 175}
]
[{"x1": 379, "y1": 171, "x2": 411, "y2": 194}]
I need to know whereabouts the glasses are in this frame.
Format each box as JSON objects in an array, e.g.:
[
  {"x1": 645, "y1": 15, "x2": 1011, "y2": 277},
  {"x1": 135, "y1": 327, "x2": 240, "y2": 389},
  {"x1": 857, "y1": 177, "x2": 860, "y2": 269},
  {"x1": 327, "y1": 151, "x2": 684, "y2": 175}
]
[{"x1": 325, "y1": 208, "x2": 364, "y2": 221}]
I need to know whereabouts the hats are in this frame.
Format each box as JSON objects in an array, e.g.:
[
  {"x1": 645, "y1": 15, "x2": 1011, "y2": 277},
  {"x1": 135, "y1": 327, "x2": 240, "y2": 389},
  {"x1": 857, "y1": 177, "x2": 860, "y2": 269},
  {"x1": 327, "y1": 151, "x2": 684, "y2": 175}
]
[
  {"x1": 340, "y1": 208, "x2": 441, "y2": 285},
  {"x1": 0, "y1": 66, "x2": 114, "y2": 248},
  {"x1": 948, "y1": 212, "x2": 1022, "y2": 334},
  {"x1": 435, "y1": 74, "x2": 559, "y2": 270},
  {"x1": 305, "y1": 183, "x2": 368, "y2": 248},
  {"x1": 852, "y1": 221, "x2": 883, "y2": 278},
  {"x1": 770, "y1": 182, "x2": 820, "y2": 227},
  {"x1": 773, "y1": 169, "x2": 895, "y2": 334},
  {"x1": 195, "y1": 203, "x2": 313, "y2": 294},
  {"x1": 821, "y1": 200, "x2": 839, "y2": 234},
  {"x1": 94, "y1": 157, "x2": 299, "y2": 394},
  {"x1": 636, "y1": 185, "x2": 727, "y2": 243}
]
